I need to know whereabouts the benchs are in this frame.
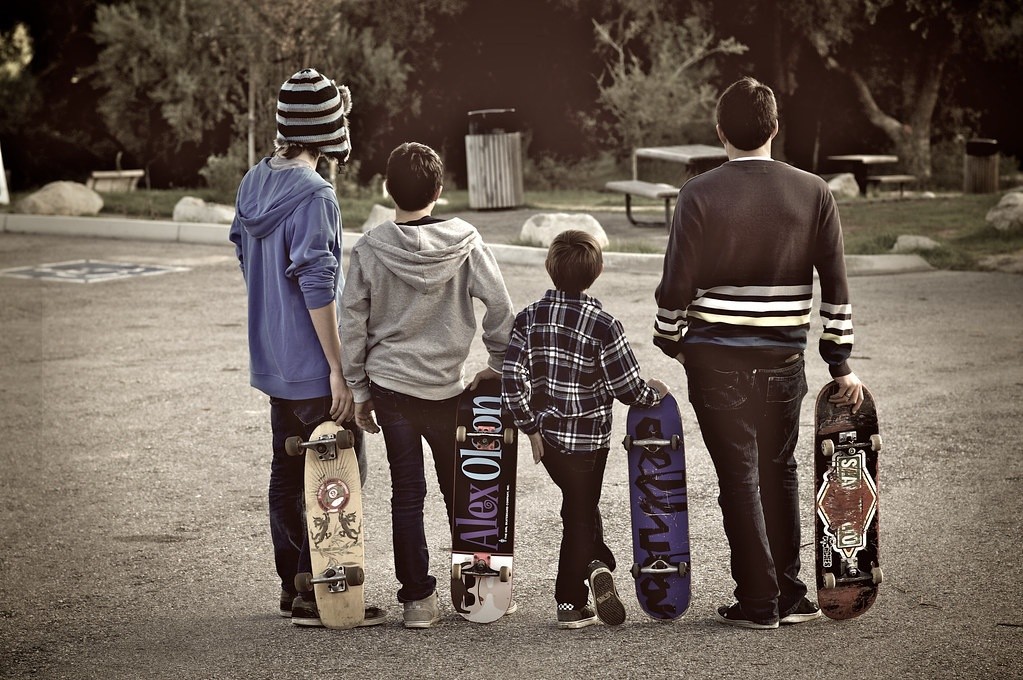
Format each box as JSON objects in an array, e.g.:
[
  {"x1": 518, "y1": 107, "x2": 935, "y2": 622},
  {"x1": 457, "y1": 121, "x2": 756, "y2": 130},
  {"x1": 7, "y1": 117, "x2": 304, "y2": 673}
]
[{"x1": 85, "y1": 169, "x2": 145, "y2": 194}]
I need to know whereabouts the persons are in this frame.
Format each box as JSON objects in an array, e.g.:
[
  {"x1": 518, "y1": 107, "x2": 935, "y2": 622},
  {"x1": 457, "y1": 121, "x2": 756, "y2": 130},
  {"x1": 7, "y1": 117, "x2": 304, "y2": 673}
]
[
  {"x1": 336, "y1": 142, "x2": 518, "y2": 629},
  {"x1": 229, "y1": 69, "x2": 388, "y2": 629},
  {"x1": 502, "y1": 231, "x2": 670, "y2": 629},
  {"x1": 652, "y1": 77, "x2": 864, "y2": 629}
]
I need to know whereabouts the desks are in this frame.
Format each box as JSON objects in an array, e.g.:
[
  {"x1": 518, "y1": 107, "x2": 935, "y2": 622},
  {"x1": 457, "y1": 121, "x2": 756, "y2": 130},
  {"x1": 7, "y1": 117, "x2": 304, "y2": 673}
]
[
  {"x1": 825, "y1": 150, "x2": 915, "y2": 203},
  {"x1": 604, "y1": 144, "x2": 731, "y2": 233}
]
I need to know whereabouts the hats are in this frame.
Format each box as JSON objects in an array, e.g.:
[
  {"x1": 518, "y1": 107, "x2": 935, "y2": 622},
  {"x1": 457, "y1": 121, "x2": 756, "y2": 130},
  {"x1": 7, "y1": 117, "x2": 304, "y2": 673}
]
[{"x1": 273, "y1": 69, "x2": 352, "y2": 166}]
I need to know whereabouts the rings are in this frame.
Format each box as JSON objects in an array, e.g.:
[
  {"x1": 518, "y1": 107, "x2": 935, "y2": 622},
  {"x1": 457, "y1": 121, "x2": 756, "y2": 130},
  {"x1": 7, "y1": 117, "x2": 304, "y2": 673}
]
[{"x1": 845, "y1": 393, "x2": 851, "y2": 398}]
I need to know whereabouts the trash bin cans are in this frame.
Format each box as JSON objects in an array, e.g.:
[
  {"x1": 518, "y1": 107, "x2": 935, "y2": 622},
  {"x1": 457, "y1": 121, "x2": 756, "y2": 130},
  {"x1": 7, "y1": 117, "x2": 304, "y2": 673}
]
[
  {"x1": 964, "y1": 137, "x2": 1000, "y2": 194},
  {"x1": 464, "y1": 109, "x2": 525, "y2": 211}
]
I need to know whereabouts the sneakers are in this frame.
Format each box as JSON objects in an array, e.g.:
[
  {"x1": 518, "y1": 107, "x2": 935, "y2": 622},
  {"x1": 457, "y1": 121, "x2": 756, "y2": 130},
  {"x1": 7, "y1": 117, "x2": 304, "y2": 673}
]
[
  {"x1": 505, "y1": 600, "x2": 516, "y2": 615},
  {"x1": 779, "y1": 596, "x2": 822, "y2": 623},
  {"x1": 557, "y1": 603, "x2": 597, "y2": 628},
  {"x1": 403, "y1": 590, "x2": 440, "y2": 627},
  {"x1": 587, "y1": 560, "x2": 626, "y2": 626},
  {"x1": 715, "y1": 602, "x2": 779, "y2": 629},
  {"x1": 276, "y1": 590, "x2": 389, "y2": 626}
]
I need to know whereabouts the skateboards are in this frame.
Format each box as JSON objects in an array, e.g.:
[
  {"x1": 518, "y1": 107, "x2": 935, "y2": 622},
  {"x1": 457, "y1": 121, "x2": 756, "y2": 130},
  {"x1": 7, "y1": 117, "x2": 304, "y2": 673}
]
[
  {"x1": 282, "y1": 419, "x2": 367, "y2": 632},
  {"x1": 810, "y1": 381, "x2": 884, "y2": 622},
  {"x1": 447, "y1": 377, "x2": 520, "y2": 624},
  {"x1": 621, "y1": 391, "x2": 693, "y2": 621}
]
